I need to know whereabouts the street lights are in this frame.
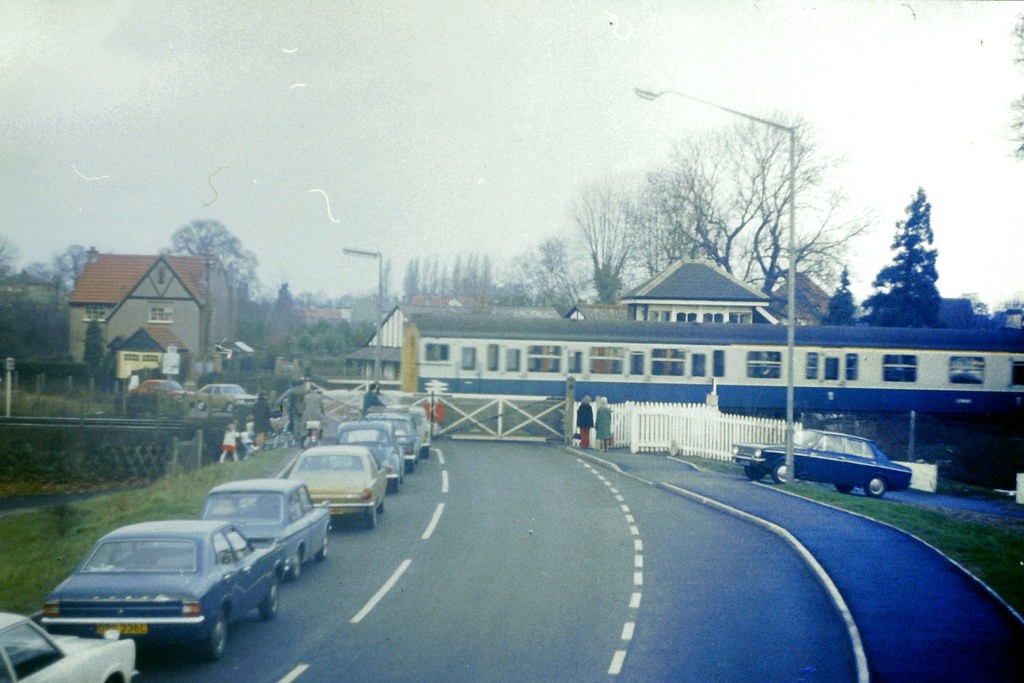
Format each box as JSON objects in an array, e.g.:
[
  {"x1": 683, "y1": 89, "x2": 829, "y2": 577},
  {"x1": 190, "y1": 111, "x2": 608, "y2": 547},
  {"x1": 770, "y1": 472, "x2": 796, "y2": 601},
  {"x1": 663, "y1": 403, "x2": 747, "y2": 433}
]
[
  {"x1": 635, "y1": 88, "x2": 795, "y2": 486},
  {"x1": 345, "y1": 248, "x2": 383, "y2": 381}
]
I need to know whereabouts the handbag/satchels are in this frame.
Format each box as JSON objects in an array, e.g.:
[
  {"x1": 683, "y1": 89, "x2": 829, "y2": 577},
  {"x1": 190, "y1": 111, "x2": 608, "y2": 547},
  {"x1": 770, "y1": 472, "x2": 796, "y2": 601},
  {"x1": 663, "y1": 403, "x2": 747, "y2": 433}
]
[
  {"x1": 607, "y1": 432, "x2": 615, "y2": 447},
  {"x1": 572, "y1": 425, "x2": 581, "y2": 441}
]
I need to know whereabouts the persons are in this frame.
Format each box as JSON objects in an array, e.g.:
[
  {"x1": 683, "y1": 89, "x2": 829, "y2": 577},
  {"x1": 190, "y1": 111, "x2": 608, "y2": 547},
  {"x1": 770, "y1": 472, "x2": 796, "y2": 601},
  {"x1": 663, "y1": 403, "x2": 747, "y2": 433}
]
[
  {"x1": 219, "y1": 423, "x2": 240, "y2": 465},
  {"x1": 577, "y1": 395, "x2": 594, "y2": 448},
  {"x1": 275, "y1": 377, "x2": 310, "y2": 447},
  {"x1": 360, "y1": 383, "x2": 386, "y2": 421},
  {"x1": 127, "y1": 370, "x2": 139, "y2": 392},
  {"x1": 251, "y1": 389, "x2": 272, "y2": 454},
  {"x1": 300, "y1": 385, "x2": 326, "y2": 449},
  {"x1": 595, "y1": 396, "x2": 612, "y2": 449},
  {"x1": 245, "y1": 410, "x2": 287, "y2": 446}
]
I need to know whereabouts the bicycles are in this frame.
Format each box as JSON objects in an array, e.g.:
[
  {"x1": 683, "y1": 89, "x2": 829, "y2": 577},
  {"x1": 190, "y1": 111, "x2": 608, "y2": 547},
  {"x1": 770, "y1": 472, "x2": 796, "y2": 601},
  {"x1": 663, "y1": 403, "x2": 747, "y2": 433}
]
[{"x1": 283, "y1": 404, "x2": 320, "y2": 450}]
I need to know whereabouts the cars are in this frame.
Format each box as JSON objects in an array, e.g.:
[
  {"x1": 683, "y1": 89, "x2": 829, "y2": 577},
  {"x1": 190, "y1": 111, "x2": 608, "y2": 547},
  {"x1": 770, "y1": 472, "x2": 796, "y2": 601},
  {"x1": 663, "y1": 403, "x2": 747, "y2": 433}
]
[
  {"x1": 386, "y1": 406, "x2": 431, "y2": 458},
  {"x1": 130, "y1": 380, "x2": 187, "y2": 402},
  {"x1": 731, "y1": 429, "x2": 912, "y2": 498},
  {"x1": 200, "y1": 478, "x2": 331, "y2": 580},
  {"x1": 363, "y1": 413, "x2": 421, "y2": 474},
  {"x1": 41, "y1": 520, "x2": 287, "y2": 661},
  {"x1": 336, "y1": 421, "x2": 405, "y2": 493},
  {"x1": 1, "y1": 613, "x2": 140, "y2": 683},
  {"x1": 287, "y1": 445, "x2": 388, "y2": 528},
  {"x1": 187, "y1": 384, "x2": 258, "y2": 413}
]
